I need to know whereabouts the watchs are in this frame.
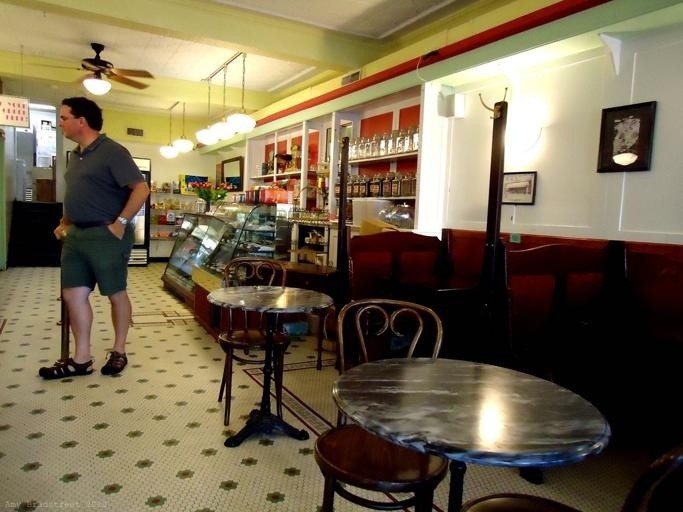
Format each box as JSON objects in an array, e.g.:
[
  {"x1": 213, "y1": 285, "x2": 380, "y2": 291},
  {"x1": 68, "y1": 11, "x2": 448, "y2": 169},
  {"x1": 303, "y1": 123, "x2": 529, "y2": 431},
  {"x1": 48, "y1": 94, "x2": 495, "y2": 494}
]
[{"x1": 116, "y1": 215, "x2": 130, "y2": 226}]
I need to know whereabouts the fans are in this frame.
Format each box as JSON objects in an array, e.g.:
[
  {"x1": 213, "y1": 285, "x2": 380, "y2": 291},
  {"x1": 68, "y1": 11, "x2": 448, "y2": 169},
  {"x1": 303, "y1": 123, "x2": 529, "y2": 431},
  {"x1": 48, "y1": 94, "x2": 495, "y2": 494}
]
[{"x1": 24, "y1": 38, "x2": 154, "y2": 94}]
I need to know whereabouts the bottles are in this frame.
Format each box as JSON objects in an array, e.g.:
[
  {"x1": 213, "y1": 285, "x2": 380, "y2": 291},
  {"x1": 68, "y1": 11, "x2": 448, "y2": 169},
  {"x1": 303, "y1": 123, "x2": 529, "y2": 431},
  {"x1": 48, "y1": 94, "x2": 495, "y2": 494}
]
[
  {"x1": 333, "y1": 170, "x2": 416, "y2": 197},
  {"x1": 287, "y1": 206, "x2": 331, "y2": 223},
  {"x1": 338, "y1": 125, "x2": 420, "y2": 160}
]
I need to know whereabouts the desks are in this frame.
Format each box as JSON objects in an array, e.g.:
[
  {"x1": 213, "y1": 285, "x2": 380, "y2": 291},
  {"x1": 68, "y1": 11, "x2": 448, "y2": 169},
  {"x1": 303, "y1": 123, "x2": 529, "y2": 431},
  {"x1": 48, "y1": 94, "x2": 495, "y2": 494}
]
[
  {"x1": 234, "y1": 255, "x2": 339, "y2": 370},
  {"x1": 206, "y1": 285, "x2": 334, "y2": 448},
  {"x1": 327, "y1": 359, "x2": 614, "y2": 510}
]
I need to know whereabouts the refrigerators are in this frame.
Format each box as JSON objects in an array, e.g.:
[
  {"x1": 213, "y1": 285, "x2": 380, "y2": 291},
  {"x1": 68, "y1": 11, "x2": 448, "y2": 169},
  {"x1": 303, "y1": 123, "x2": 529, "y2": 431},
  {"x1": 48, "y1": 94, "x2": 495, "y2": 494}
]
[{"x1": 119, "y1": 150, "x2": 150, "y2": 266}]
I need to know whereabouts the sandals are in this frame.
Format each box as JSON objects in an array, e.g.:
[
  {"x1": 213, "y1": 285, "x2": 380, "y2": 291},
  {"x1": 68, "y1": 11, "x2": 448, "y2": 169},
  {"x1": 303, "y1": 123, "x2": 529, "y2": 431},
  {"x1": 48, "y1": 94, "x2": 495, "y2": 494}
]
[
  {"x1": 101, "y1": 351, "x2": 127, "y2": 374},
  {"x1": 39, "y1": 358, "x2": 92, "y2": 379}
]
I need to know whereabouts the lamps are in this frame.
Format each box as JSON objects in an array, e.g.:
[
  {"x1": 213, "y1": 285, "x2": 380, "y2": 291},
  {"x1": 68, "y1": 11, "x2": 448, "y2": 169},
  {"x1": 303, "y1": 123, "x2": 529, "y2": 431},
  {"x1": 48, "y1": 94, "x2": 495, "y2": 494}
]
[
  {"x1": 152, "y1": 98, "x2": 192, "y2": 162},
  {"x1": 80, "y1": 71, "x2": 112, "y2": 97},
  {"x1": 191, "y1": 52, "x2": 256, "y2": 154}
]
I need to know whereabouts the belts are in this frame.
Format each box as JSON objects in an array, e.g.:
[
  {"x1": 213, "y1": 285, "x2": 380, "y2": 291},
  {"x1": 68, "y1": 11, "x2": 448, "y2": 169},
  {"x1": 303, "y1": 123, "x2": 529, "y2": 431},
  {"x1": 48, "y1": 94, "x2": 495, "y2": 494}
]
[{"x1": 73, "y1": 221, "x2": 114, "y2": 228}]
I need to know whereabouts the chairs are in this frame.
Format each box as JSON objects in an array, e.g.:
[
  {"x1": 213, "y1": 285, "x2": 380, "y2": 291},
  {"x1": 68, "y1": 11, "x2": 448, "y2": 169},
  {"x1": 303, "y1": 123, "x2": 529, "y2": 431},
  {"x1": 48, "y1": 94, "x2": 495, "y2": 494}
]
[
  {"x1": 311, "y1": 297, "x2": 450, "y2": 509},
  {"x1": 216, "y1": 255, "x2": 295, "y2": 426},
  {"x1": 455, "y1": 445, "x2": 683, "y2": 512}
]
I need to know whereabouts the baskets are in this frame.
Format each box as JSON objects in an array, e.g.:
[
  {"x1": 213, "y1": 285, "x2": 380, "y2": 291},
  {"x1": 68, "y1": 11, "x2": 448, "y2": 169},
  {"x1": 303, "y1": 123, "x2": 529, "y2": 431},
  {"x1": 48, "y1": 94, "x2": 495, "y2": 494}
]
[{"x1": 293, "y1": 186, "x2": 330, "y2": 225}]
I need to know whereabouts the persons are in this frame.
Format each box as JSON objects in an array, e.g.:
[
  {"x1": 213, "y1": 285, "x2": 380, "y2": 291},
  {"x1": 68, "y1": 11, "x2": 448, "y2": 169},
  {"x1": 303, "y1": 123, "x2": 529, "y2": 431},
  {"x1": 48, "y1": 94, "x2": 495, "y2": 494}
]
[{"x1": 37, "y1": 93, "x2": 151, "y2": 380}]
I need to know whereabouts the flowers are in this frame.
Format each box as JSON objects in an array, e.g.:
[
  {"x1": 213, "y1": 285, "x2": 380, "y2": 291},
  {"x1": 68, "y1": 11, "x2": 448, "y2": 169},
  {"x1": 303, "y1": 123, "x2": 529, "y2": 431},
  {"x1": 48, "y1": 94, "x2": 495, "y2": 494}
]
[{"x1": 189, "y1": 180, "x2": 234, "y2": 203}]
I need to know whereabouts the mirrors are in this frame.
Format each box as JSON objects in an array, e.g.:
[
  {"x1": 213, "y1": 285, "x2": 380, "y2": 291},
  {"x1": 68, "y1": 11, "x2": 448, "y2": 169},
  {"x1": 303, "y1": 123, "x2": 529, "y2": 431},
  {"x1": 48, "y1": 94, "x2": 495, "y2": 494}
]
[{"x1": 221, "y1": 156, "x2": 243, "y2": 193}]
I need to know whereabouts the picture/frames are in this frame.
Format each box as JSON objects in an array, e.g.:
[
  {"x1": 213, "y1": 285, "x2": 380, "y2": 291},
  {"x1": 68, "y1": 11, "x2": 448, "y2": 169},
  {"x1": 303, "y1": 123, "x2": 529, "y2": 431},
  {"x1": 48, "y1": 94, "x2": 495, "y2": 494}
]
[
  {"x1": 593, "y1": 100, "x2": 658, "y2": 175},
  {"x1": 498, "y1": 170, "x2": 538, "y2": 207}
]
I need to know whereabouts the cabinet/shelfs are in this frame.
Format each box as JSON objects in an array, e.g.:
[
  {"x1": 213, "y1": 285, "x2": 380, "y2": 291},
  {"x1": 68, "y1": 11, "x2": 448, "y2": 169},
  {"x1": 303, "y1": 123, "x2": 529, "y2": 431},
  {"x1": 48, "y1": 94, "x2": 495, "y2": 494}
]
[
  {"x1": 288, "y1": 217, "x2": 339, "y2": 268},
  {"x1": 150, "y1": 192, "x2": 196, "y2": 244},
  {"x1": 248, "y1": 169, "x2": 316, "y2": 205},
  {"x1": 160, "y1": 203, "x2": 278, "y2": 308},
  {"x1": 330, "y1": 149, "x2": 417, "y2": 233}
]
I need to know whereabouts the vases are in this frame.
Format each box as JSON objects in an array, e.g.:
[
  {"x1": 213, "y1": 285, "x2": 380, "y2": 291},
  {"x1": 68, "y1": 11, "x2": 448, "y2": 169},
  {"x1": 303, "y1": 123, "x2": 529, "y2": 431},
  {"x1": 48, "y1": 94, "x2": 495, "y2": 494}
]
[{"x1": 200, "y1": 200, "x2": 212, "y2": 212}]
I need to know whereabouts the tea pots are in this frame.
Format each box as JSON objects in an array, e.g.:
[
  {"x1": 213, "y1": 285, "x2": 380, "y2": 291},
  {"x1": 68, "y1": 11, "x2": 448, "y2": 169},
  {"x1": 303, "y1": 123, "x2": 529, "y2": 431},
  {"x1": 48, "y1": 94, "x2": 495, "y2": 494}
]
[{"x1": 377, "y1": 202, "x2": 413, "y2": 228}]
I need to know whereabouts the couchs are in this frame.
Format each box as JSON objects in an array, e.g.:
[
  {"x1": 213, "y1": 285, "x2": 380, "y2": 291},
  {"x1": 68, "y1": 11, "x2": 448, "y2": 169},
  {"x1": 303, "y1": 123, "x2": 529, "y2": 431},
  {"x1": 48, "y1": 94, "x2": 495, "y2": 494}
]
[{"x1": 340, "y1": 226, "x2": 678, "y2": 382}]
